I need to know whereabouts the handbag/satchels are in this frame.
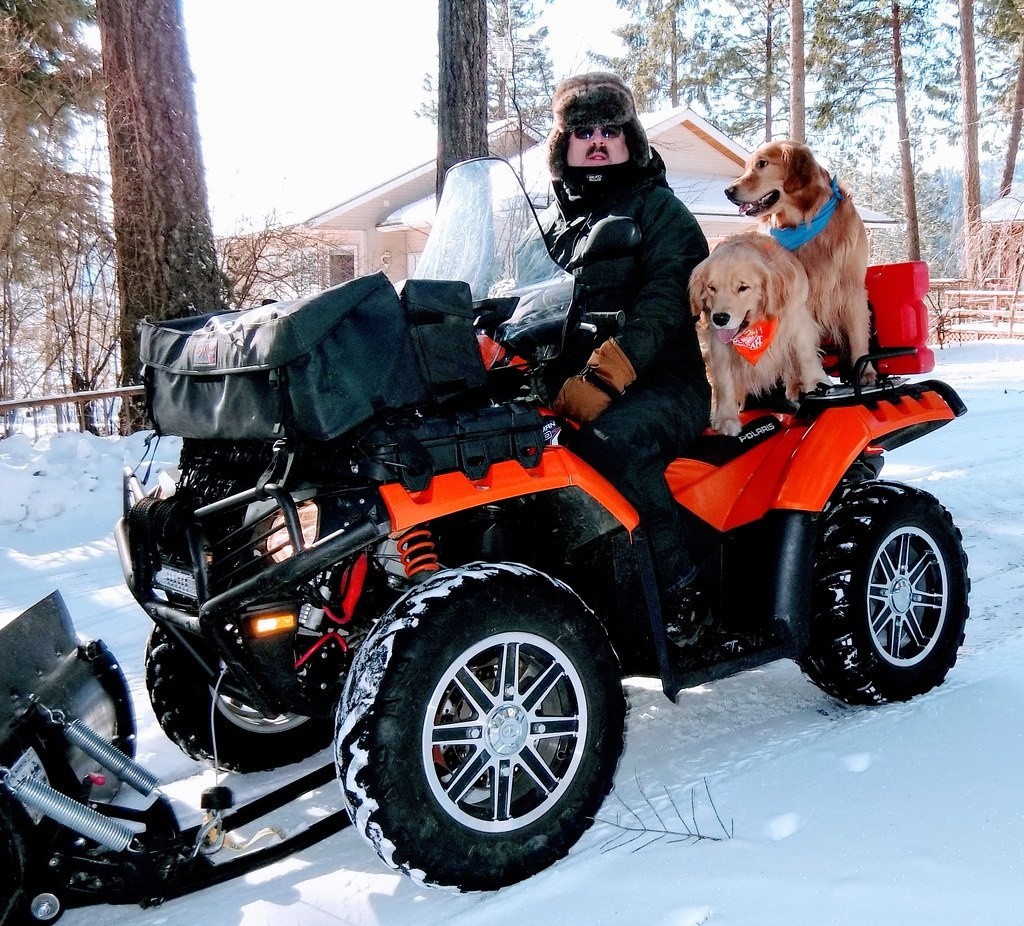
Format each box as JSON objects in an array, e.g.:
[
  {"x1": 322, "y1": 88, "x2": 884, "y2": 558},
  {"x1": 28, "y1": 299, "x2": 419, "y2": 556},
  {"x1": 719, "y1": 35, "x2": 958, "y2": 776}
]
[
  {"x1": 393, "y1": 278, "x2": 491, "y2": 418},
  {"x1": 139, "y1": 270, "x2": 427, "y2": 447}
]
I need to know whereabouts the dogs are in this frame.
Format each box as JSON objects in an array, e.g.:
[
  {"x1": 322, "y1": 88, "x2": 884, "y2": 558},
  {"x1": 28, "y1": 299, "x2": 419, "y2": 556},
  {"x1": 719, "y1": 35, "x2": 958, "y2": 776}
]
[{"x1": 688, "y1": 138, "x2": 878, "y2": 436}]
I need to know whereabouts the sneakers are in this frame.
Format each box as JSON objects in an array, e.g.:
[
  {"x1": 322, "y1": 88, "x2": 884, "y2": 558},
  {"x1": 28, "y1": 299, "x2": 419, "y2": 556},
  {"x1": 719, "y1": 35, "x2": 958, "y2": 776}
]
[{"x1": 663, "y1": 574, "x2": 714, "y2": 648}]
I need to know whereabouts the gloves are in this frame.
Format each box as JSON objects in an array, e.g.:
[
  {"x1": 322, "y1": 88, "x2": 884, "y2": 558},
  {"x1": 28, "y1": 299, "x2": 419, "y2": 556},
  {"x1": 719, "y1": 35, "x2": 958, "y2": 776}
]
[{"x1": 549, "y1": 336, "x2": 637, "y2": 423}]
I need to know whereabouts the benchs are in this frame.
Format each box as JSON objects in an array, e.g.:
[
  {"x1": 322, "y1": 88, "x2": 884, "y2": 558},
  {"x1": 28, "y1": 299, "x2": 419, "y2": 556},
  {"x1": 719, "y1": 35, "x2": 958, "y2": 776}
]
[{"x1": 807, "y1": 262, "x2": 934, "y2": 409}]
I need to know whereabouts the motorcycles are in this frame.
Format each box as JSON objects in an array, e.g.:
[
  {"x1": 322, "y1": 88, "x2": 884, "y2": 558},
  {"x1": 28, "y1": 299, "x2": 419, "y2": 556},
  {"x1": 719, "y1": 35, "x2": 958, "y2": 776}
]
[{"x1": 113, "y1": 155, "x2": 969, "y2": 926}]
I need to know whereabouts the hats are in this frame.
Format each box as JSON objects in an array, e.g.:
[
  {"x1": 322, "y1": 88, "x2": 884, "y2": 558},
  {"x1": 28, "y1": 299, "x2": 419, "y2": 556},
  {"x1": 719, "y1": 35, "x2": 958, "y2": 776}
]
[{"x1": 545, "y1": 72, "x2": 650, "y2": 180}]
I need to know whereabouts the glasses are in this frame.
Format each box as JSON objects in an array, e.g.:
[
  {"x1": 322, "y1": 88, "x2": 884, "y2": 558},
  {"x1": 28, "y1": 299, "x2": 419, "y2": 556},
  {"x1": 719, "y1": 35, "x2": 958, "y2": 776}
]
[{"x1": 568, "y1": 124, "x2": 625, "y2": 140}]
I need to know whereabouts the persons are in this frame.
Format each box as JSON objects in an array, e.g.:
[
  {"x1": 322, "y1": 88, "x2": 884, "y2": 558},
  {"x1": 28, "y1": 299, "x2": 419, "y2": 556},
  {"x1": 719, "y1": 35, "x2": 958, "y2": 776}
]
[{"x1": 489, "y1": 73, "x2": 714, "y2": 647}]
